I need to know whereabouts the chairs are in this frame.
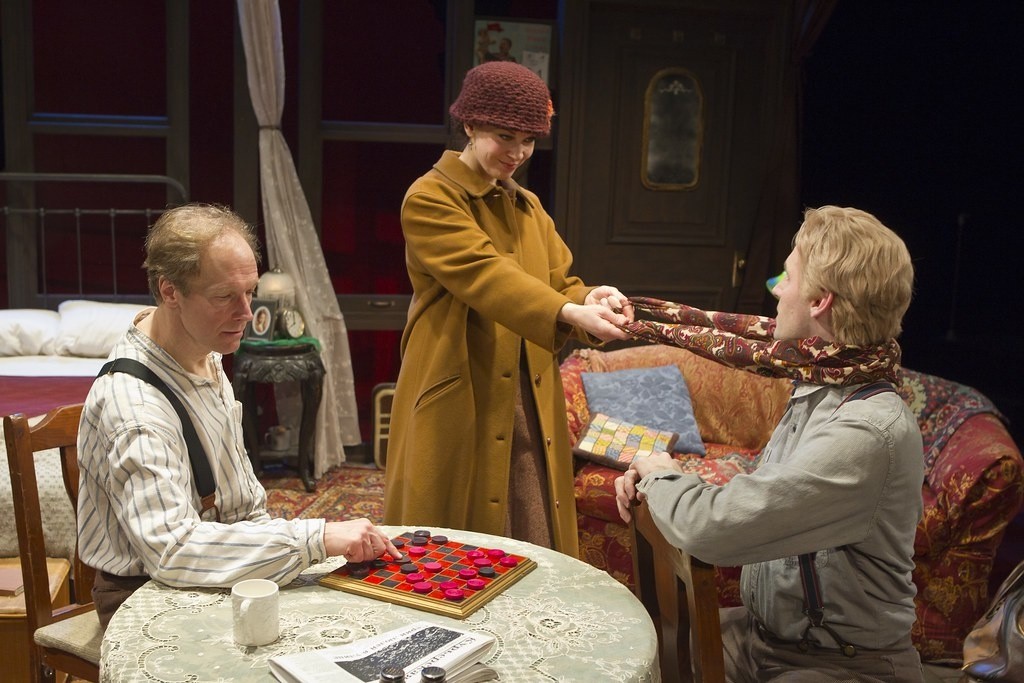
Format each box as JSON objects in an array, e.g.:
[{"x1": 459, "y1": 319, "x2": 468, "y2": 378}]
[
  {"x1": 3, "y1": 402, "x2": 104, "y2": 683},
  {"x1": 628, "y1": 494, "x2": 724, "y2": 683}
]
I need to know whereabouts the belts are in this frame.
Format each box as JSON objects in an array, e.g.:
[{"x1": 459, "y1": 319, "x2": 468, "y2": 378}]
[{"x1": 748, "y1": 610, "x2": 806, "y2": 645}]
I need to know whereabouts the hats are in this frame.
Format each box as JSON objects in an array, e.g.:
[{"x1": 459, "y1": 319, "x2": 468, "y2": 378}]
[{"x1": 451, "y1": 62, "x2": 552, "y2": 134}]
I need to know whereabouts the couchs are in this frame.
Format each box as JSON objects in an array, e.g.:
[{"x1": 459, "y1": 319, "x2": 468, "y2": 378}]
[{"x1": 560, "y1": 345, "x2": 1024, "y2": 660}]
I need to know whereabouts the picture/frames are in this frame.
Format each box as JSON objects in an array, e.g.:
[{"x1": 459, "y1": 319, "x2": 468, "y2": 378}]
[{"x1": 243, "y1": 298, "x2": 279, "y2": 342}]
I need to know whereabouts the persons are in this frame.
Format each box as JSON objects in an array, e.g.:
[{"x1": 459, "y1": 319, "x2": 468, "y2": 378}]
[
  {"x1": 474, "y1": 28, "x2": 517, "y2": 63},
  {"x1": 380, "y1": 62, "x2": 634, "y2": 560},
  {"x1": 76, "y1": 206, "x2": 403, "y2": 635},
  {"x1": 612, "y1": 206, "x2": 923, "y2": 683},
  {"x1": 255, "y1": 310, "x2": 267, "y2": 333}
]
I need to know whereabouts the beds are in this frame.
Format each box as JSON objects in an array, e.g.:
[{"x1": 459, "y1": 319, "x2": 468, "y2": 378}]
[{"x1": 0, "y1": 173, "x2": 191, "y2": 605}]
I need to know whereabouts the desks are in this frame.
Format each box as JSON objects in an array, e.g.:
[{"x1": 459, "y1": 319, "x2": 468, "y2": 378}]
[
  {"x1": 99, "y1": 526, "x2": 662, "y2": 683},
  {"x1": 230, "y1": 342, "x2": 327, "y2": 492}
]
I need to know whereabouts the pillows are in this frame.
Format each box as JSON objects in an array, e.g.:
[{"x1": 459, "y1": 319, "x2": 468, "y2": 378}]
[
  {"x1": 55, "y1": 298, "x2": 148, "y2": 358},
  {"x1": 0, "y1": 308, "x2": 60, "y2": 357},
  {"x1": 580, "y1": 366, "x2": 707, "y2": 457}
]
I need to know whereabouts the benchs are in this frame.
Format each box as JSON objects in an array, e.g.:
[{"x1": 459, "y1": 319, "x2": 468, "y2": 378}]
[{"x1": 0, "y1": 556, "x2": 72, "y2": 683}]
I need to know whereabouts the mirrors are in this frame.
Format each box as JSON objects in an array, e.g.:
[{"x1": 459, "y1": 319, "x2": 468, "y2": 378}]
[{"x1": 639, "y1": 70, "x2": 708, "y2": 192}]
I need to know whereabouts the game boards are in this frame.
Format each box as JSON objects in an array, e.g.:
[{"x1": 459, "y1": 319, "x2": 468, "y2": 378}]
[{"x1": 316, "y1": 531, "x2": 539, "y2": 621}]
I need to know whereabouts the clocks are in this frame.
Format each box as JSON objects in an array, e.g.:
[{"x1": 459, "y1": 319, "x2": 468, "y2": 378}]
[{"x1": 277, "y1": 307, "x2": 306, "y2": 340}]
